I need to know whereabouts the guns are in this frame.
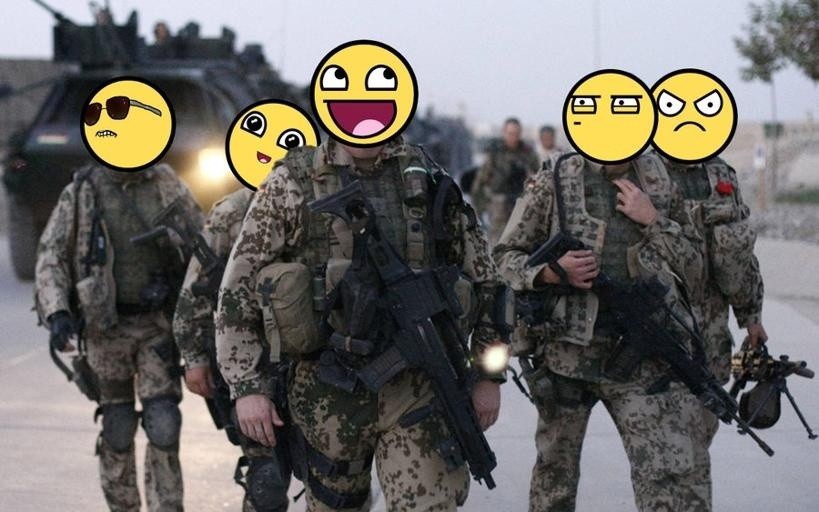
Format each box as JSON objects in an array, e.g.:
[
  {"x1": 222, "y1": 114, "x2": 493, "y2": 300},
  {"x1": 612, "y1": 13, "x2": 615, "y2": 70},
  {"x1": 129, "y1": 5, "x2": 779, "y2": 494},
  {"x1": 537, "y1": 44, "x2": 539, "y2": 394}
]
[
  {"x1": 151, "y1": 196, "x2": 226, "y2": 310},
  {"x1": 129, "y1": 224, "x2": 186, "y2": 361},
  {"x1": 728, "y1": 336, "x2": 818, "y2": 438},
  {"x1": 522, "y1": 230, "x2": 774, "y2": 457},
  {"x1": 307, "y1": 177, "x2": 497, "y2": 490}
]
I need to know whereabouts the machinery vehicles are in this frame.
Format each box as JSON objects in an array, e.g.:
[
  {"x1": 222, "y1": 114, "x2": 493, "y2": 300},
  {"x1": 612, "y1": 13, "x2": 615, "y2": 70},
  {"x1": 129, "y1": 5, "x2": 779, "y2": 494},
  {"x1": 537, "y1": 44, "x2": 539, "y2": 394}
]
[
  {"x1": 0, "y1": 1, "x2": 307, "y2": 281},
  {"x1": 294, "y1": 97, "x2": 473, "y2": 189}
]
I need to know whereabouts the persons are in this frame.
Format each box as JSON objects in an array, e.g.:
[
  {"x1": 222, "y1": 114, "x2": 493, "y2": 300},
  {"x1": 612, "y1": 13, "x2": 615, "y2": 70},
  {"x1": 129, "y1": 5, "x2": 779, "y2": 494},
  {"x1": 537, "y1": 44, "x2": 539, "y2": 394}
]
[
  {"x1": 210, "y1": 132, "x2": 514, "y2": 512},
  {"x1": 29, "y1": 158, "x2": 211, "y2": 511},
  {"x1": 152, "y1": 22, "x2": 171, "y2": 48},
  {"x1": 94, "y1": 9, "x2": 115, "y2": 28},
  {"x1": 489, "y1": 151, "x2": 716, "y2": 511},
  {"x1": 534, "y1": 124, "x2": 569, "y2": 169},
  {"x1": 470, "y1": 116, "x2": 539, "y2": 253},
  {"x1": 646, "y1": 148, "x2": 770, "y2": 446},
  {"x1": 173, "y1": 183, "x2": 294, "y2": 511}
]
